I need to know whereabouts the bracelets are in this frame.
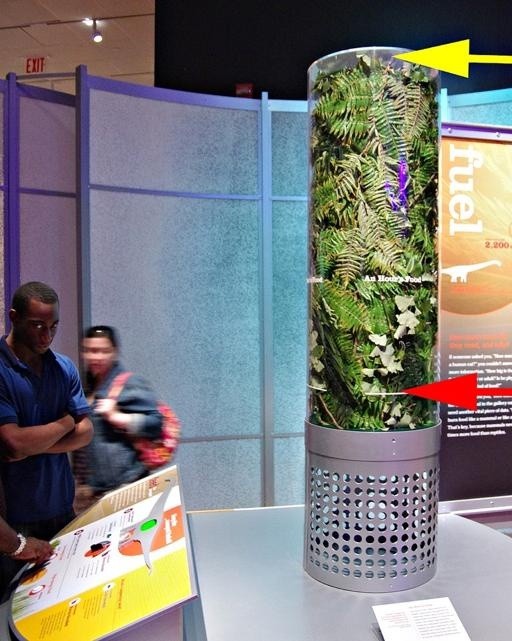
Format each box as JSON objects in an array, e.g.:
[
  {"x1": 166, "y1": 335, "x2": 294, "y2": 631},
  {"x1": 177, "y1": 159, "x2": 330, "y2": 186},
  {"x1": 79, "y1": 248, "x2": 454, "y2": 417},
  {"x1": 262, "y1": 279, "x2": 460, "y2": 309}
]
[{"x1": 5, "y1": 533, "x2": 27, "y2": 557}]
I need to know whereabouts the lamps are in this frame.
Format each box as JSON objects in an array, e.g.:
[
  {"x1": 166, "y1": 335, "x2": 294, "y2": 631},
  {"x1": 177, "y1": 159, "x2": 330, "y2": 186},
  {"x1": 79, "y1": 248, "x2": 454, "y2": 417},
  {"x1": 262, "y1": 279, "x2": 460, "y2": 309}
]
[{"x1": 90, "y1": 17, "x2": 102, "y2": 42}]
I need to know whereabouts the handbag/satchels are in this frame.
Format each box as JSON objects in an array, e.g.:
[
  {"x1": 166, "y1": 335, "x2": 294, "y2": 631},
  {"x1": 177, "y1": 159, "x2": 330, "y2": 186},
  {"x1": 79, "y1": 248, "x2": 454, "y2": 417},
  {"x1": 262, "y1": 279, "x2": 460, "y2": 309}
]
[{"x1": 130, "y1": 404, "x2": 180, "y2": 470}]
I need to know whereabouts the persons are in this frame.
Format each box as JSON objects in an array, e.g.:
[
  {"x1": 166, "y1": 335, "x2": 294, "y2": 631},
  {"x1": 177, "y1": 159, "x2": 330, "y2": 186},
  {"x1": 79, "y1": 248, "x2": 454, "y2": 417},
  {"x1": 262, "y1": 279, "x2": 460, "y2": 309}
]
[
  {"x1": 66, "y1": 325, "x2": 162, "y2": 517},
  {"x1": 0, "y1": 280, "x2": 95, "y2": 541},
  {"x1": 0, "y1": 517, "x2": 53, "y2": 565}
]
